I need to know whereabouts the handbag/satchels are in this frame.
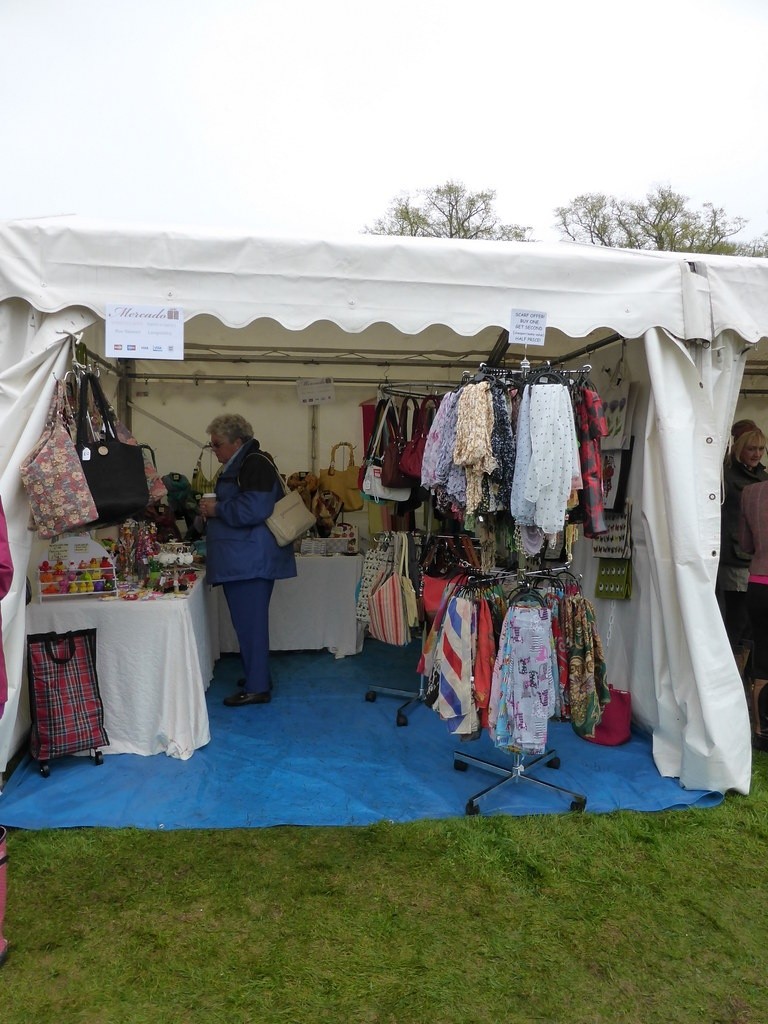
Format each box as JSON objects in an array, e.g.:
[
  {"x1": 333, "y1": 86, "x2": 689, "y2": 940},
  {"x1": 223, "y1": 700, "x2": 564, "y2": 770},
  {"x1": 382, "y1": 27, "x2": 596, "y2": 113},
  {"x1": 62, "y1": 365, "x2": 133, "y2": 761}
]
[
  {"x1": 18, "y1": 373, "x2": 168, "y2": 540},
  {"x1": 25, "y1": 628, "x2": 110, "y2": 778},
  {"x1": 237, "y1": 453, "x2": 316, "y2": 548},
  {"x1": 593, "y1": 343, "x2": 640, "y2": 601},
  {"x1": 356, "y1": 530, "x2": 481, "y2": 647},
  {"x1": 194, "y1": 444, "x2": 224, "y2": 493},
  {"x1": 581, "y1": 684, "x2": 631, "y2": 745},
  {"x1": 320, "y1": 442, "x2": 364, "y2": 512},
  {"x1": 544, "y1": 527, "x2": 565, "y2": 562},
  {"x1": 329, "y1": 523, "x2": 358, "y2": 554},
  {"x1": 357, "y1": 394, "x2": 441, "y2": 517}
]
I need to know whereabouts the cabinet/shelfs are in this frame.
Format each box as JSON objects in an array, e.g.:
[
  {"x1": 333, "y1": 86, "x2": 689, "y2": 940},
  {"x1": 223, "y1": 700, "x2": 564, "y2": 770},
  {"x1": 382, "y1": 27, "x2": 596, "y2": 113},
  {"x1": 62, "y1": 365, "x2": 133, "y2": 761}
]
[{"x1": 36, "y1": 564, "x2": 120, "y2": 604}]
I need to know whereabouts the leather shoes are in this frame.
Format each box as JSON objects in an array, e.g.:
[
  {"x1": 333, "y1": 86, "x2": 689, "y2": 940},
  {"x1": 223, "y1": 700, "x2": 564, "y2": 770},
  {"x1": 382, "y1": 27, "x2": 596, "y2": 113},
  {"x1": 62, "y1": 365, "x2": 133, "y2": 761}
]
[
  {"x1": 239, "y1": 677, "x2": 247, "y2": 687},
  {"x1": 223, "y1": 691, "x2": 271, "y2": 706}
]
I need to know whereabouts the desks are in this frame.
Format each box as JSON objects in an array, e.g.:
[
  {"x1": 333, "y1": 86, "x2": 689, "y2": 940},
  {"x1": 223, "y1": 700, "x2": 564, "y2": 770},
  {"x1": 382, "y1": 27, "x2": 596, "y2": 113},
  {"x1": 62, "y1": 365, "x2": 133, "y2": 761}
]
[
  {"x1": 219, "y1": 553, "x2": 365, "y2": 660},
  {"x1": 26, "y1": 565, "x2": 219, "y2": 760}
]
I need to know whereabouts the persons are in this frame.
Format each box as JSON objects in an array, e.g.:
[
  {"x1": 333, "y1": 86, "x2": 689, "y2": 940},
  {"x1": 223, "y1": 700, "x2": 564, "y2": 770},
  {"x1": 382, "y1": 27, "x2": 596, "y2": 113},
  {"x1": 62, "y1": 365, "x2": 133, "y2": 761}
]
[
  {"x1": 738, "y1": 480, "x2": 768, "y2": 752},
  {"x1": 716, "y1": 420, "x2": 768, "y2": 673},
  {"x1": 198, "y1": 414, "x2": 297, "y2": 708}
]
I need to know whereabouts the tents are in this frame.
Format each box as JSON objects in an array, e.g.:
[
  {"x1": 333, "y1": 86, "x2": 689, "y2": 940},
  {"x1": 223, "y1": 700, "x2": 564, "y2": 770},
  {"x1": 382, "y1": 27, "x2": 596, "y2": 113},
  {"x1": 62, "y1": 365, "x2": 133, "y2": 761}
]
[{"x1": 0, "y1": 218, "x2": 768, "y2": 808}]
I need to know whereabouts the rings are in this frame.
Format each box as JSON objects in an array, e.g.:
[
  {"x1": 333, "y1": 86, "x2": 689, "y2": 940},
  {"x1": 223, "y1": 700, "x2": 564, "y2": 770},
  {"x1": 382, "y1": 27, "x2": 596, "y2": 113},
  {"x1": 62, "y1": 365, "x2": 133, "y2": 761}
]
[{"x1": 203, "y1": 505, "x2": 206, "y2": 508}]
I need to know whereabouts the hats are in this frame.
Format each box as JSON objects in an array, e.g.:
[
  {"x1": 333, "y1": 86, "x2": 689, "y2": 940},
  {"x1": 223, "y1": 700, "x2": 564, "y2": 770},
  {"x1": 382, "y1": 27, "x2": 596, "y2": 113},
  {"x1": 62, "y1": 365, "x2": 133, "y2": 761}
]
[{"x1": 731, "y1": 419, "x2": 762, "y2": 443}]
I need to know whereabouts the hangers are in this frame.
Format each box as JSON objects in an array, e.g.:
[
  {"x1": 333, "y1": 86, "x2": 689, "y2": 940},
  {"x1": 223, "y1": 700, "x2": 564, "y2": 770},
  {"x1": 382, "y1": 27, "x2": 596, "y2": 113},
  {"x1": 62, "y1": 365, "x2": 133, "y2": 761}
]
[
  {"x1": 458, "y1": 578, "x2": 581, "y2": 609},
  {"x1": 464, "y1": 365, "x2": 598, "y2": 390}
]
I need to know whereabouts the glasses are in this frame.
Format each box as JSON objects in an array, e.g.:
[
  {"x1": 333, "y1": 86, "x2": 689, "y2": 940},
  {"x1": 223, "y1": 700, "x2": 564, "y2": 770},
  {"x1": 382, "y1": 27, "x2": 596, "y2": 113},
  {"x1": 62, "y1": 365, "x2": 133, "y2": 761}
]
[{"x1": 209, "y1": 439, "x2": 226, "y2": 448}]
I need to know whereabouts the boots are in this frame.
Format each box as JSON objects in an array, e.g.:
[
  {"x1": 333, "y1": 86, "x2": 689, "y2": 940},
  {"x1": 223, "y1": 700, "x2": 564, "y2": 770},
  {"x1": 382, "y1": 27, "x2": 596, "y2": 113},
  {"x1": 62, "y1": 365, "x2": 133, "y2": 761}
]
[
  {"x1": 734, "y1": 645, "x2": 751, "y2": 711},
  {"x1": 752, "y1": 679, "x2": 767, "y2": 752}
]
